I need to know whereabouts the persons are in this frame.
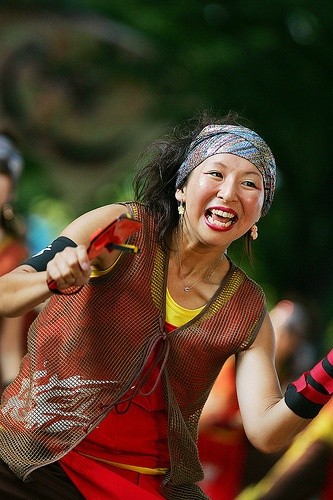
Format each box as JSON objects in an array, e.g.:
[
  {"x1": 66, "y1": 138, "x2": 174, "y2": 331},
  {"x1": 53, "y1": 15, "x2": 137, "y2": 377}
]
[
  {"x1": 192, "y1": 300, "x2": 306, "y2": 500},
  {"x1": 0, "y1": 106, "x2": 333, "y2": 500},
  {"x1": 0, "y1": 134, "x2": 50, "y2": 392}
]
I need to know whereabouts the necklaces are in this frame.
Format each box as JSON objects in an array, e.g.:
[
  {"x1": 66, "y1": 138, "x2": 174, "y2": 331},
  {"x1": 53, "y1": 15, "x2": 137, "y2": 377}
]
[{"x1": 175, "y1": 227, "x2": 223, "y2": 292}]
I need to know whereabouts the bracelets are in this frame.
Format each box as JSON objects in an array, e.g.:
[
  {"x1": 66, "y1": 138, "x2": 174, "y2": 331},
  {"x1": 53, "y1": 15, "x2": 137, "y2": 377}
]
[{"x1": 46, "y1": 276, "x2": 85, "y2": 295}]
